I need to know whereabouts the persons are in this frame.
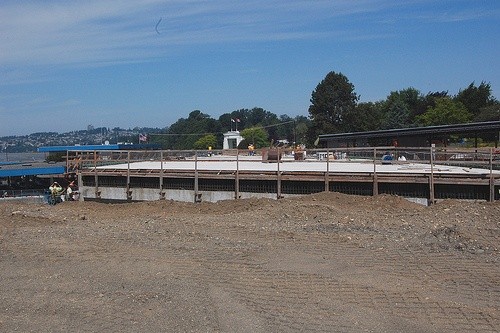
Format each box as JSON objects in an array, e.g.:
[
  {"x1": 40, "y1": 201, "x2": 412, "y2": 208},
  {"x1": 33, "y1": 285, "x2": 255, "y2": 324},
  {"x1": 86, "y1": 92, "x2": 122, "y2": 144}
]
[
  {"x1": 67, "y1": 184, "x2": 75, "y2": 200},
  {"x1": 248, "y1": 144, "x2": 255, "y2": 155},
  {"x1": 207, "y1": 145, "x2": 212, "y2": 156},
  {"x1": 48, "y1": 181, "x2": 62, "y2": 202}
]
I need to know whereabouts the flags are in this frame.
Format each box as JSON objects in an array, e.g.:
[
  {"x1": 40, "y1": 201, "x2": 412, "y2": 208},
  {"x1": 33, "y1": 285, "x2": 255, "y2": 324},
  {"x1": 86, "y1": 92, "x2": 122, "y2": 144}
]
[
  {"x1": 231, "y1": 118, "x2": 235, "y2": 123},
  {"x1": 236, "y1": 118, "x2": 240, "y2": 123}
]
